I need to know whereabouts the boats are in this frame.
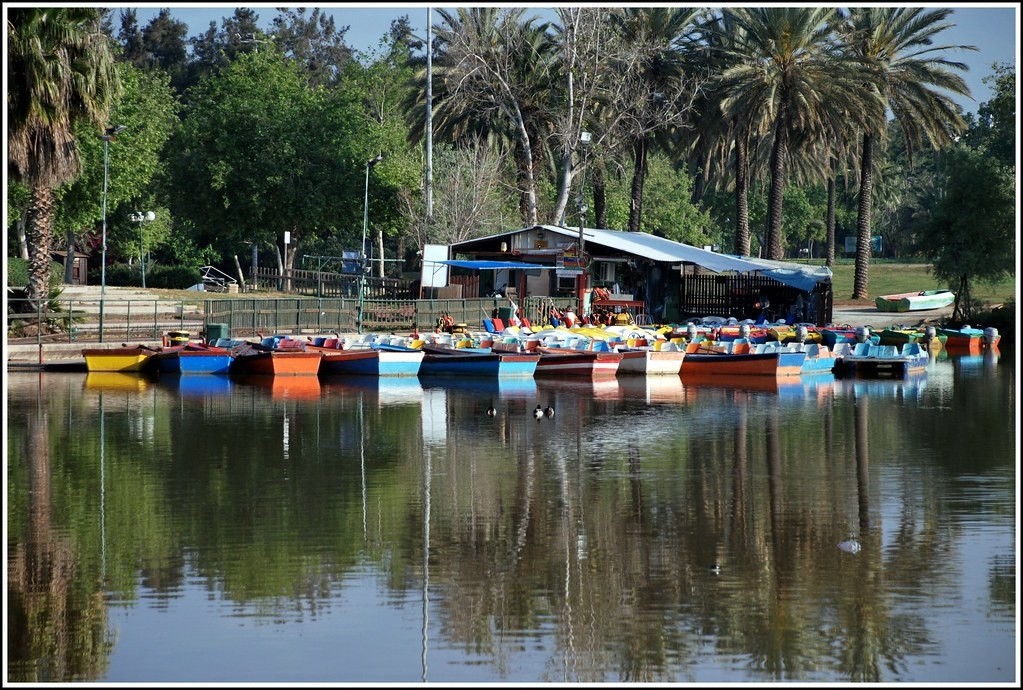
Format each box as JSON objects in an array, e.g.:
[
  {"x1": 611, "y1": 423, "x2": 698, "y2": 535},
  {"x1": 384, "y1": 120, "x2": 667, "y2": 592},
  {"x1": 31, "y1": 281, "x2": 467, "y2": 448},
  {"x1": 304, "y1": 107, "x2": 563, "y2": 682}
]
[
  {"x1": 156, "y1": 370, "x2": 230, "y2": 395},
  {"x1": 878, "y1": 330, "x2": 949, "y2": 349},
  {"x1": 234, "y1": 349, "x2": 325, "y2": 377},
  {"x1": 618, "y1": 350, "x2": 686, "y2": 374},
  {"x1": 82, "y1": 371, "x2": 151, "y2": 393},
  {"x1": 300, "y1": 343, "x2": 428, "y2": 375},
  {"x1": 237, "y1": 374, "x2": 322, "y2": 400},
  {"x1": 799, "y1": 355, "x2": 839, "y2": 374},
  {"x1": 321, "y1": 373, "x2": 424, "y2": 400},
  {"x1": 613, "y1": 373, "x2": 686, "y2": 403},
  {"x1": 419, "y1": 373, "x2": 540, "y2": 397},
  {"x1": 678, "y1": 370, "x2": 838, "y2": 397},
  {"x1": 680, "y1": 350, "x2": 807, "y2": 375},
  {"x1": 944, "y1": 343, "x2": 1003, "y2": 367},
  {"x1": 478, "y1": 338, "x2": 624, "y2": 376},
  {"x1": 938, "y1": 327, "x2": 1002, "y2": 349},
  {"x1": 418, "y1": 347, "x2": 544, "y2": 376},
  {"x1": 80, "y1": 345, "x2": 158, "y2": 373},
  {"x1": 874, "y1": 288, "x2": 958, "y2": 313},
  {"x1": 533, "y1": 373, "x2": 621, "y2": 398},
  {"x1": 157, "y1": 350, "x2": 233, "y2": 375}
]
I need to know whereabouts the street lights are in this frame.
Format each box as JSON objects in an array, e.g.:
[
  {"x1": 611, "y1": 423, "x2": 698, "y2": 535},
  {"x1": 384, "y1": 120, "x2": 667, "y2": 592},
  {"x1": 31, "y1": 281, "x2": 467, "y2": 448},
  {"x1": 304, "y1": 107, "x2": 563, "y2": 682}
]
[
  {"x1": 96, "y1": 123, "x2": 130, "y2": 344},
  {"x1": 126, "y1": 212, "x2": 156, "y2": 290},
  {"x1": 362, "y1": 153, "x2": 384, "y2": 258}
]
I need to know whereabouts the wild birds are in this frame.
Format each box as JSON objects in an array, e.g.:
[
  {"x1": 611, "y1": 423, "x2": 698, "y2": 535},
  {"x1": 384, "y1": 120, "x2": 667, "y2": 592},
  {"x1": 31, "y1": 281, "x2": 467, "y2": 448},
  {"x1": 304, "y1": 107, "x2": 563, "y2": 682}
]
[
  {"x1": 546, "y1": 406, "x2": 554, "y2": 419},
  {"x1": 486, "y1": 405, "x2": 497, "y2": 418},
  {"x1": 534, "y1": 405, "x2": 544, "y2": 421}
]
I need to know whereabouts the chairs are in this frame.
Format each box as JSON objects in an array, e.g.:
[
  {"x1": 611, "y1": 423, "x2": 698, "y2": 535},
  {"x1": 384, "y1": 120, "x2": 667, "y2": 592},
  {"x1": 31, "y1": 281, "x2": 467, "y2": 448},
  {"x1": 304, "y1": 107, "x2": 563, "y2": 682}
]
[{"x1": 260, "y1": 314, "x2": 928, "y2": 358}]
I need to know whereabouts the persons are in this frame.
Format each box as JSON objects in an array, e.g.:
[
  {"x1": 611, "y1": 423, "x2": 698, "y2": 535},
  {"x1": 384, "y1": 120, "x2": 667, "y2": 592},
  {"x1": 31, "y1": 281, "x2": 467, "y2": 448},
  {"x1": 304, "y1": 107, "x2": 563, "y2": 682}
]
[
  {"x1": 435, "y1": 312, "x2": 454, "y2": 335},
  {"x1": 566, "y1": 305, "x2": 576, "y2": 324},
  {"x1": 508, "y1": 298, "x2": 522, "y2": 328}
]
